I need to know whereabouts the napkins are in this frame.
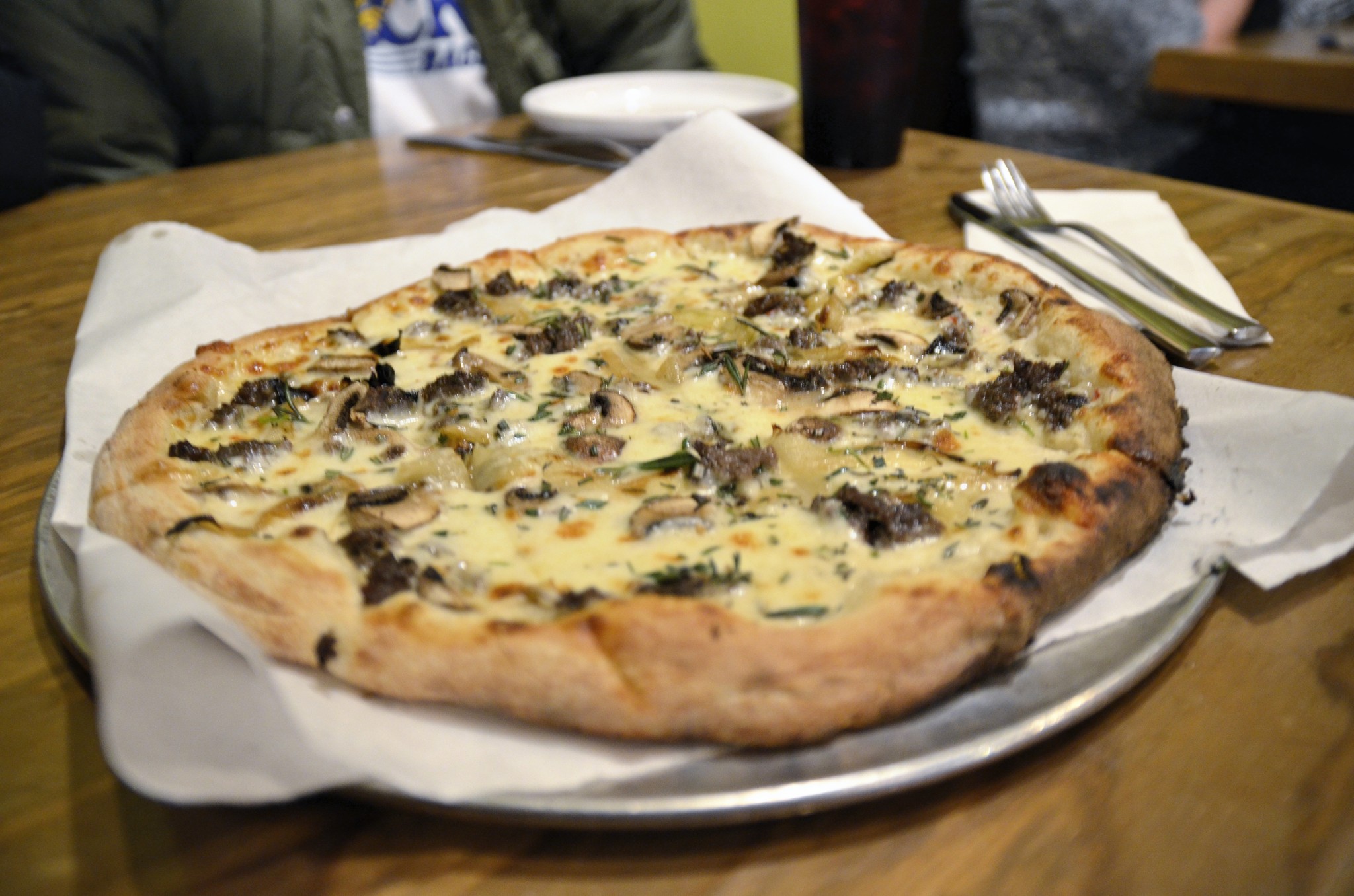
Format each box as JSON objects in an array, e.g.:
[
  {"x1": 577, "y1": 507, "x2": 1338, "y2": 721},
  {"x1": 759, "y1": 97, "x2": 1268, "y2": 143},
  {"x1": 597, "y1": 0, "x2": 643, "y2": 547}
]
[{"x1": 958, "y1": 188, "x2": 1275, "y2": 347}]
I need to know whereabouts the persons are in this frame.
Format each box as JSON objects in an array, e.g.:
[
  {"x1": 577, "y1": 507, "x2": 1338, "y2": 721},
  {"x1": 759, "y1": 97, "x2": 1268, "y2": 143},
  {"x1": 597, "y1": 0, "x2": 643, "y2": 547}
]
[
  {"x1": 1194, "y1": 0, "x2": 1354, "y2": 215},
  {"x1": 1, "y1": 0, "x2": 718, "y2": 218}
]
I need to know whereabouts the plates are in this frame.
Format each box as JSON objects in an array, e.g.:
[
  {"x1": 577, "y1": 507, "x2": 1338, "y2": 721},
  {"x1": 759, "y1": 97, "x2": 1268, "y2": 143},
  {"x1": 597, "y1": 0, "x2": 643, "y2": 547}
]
[
  {"x1": 34, "y1": 459, "x2": 1232, "y2": 825},
  {"x1": 517, "y1": 70, "x2": 798, "y2": 148}
]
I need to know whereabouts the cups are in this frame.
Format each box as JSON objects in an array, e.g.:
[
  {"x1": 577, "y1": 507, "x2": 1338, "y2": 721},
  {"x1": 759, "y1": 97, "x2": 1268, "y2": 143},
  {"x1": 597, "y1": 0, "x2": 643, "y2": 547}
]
[{"x1": 797, "y1": 0, "x2": 911, "y2": 167}]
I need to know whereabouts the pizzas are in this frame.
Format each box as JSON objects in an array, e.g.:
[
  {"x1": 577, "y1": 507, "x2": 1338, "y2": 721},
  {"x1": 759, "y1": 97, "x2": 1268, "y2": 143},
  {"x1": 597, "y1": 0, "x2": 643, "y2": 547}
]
[{"x1": 86, "y1": 214, "x2": 1186, "y2": 746}]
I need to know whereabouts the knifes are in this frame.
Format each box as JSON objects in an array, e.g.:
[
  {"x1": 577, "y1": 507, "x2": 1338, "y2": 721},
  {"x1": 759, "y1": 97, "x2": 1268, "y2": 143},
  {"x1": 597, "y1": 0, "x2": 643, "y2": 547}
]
[
  {"x1": 404, "y1": 132, "x2": 626, "y2": 171},
  {"x1": 953, "y1": 190, "x2": 1225, "y2": 365}
]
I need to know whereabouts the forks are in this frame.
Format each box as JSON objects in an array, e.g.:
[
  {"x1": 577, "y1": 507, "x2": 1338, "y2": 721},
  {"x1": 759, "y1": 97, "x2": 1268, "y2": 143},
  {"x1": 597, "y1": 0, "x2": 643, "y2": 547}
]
[{"x1": 979, "y1": 158, "x2": 1269, "y2": 342}]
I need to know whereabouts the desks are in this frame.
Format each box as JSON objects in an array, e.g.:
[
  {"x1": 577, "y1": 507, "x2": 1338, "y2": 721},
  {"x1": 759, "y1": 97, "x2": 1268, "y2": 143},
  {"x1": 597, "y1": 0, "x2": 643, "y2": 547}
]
[{"x1": 1, "y1": 119, "x2": 1354, "y2": 896}]
